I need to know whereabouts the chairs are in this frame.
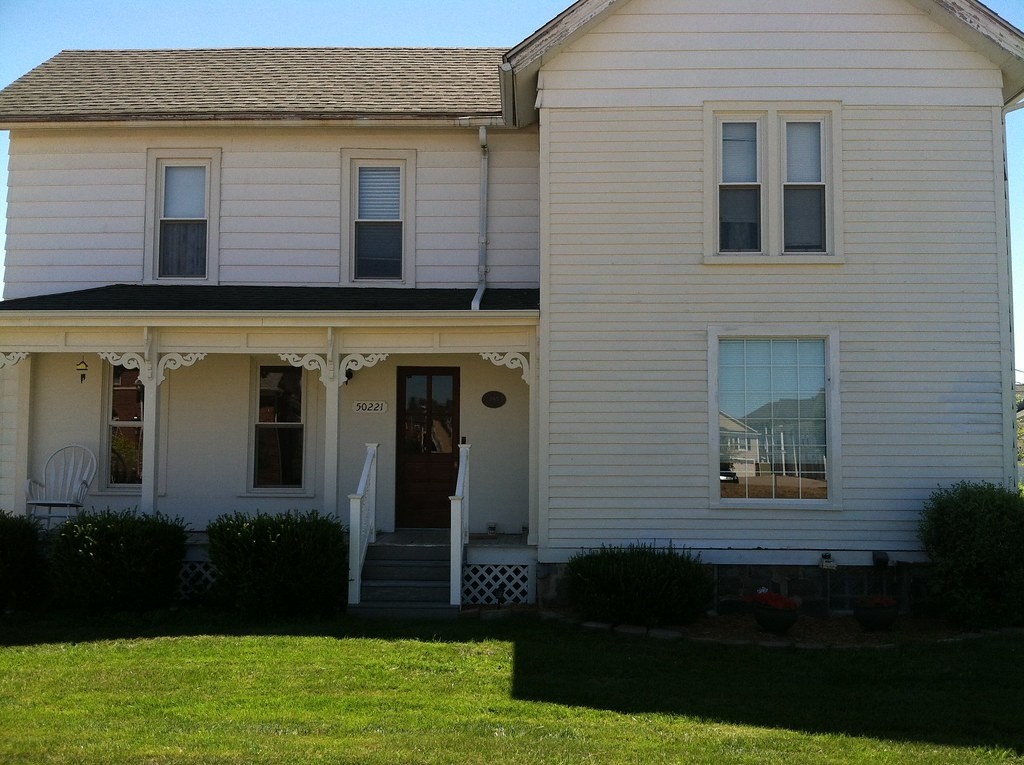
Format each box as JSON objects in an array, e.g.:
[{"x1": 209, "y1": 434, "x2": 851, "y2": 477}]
[{"x1": 24, "y1": 444, "x2": 98, "y2": 529}]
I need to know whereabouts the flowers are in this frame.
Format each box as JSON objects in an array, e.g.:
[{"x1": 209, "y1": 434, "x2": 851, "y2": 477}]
[
  {"x1": 756, "y1": 587, "x2": 802, "y2": 610},
  {"x1": 857, "y1": 597, "x2": 897, "y2": 606}
]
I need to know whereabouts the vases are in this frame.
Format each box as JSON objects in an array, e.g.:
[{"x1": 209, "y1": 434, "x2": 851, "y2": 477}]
[
  {"x1": 754, "y1": 604, "x2": 798, "y2": 633},
  {"x1": 855, "y1": 605, "x2": 897, "y2": 628}
]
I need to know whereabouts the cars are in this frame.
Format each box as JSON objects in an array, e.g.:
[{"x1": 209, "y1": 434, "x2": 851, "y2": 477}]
[{"x1": 720, "y1": 467, "x2": 740, "y2": 484}]
